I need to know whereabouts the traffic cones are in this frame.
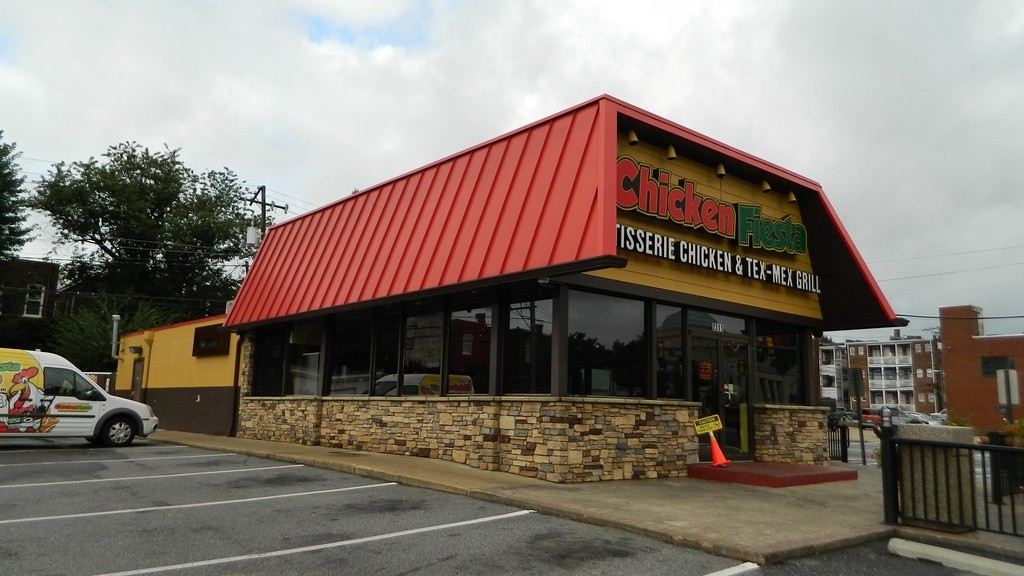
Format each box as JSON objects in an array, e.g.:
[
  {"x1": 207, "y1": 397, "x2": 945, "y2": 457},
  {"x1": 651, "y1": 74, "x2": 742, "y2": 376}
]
[{"x1": 708, "y1": 431, "x2": 731, "y2": 468}]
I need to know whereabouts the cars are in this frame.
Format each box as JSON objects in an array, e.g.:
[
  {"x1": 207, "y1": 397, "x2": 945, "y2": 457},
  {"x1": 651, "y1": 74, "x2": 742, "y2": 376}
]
[{"x1": 827, "y1": 404, "x2": 947, "y2": 439}]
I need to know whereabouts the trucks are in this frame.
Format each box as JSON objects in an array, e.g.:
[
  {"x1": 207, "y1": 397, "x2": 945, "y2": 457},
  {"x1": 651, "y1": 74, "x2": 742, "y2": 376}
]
[
  {"x1": 362, "y1": 373, "x2": 474, "y2": 396},
  {"x1": 0, "y1": 348, "x2": 158, "y2": 447}
]
